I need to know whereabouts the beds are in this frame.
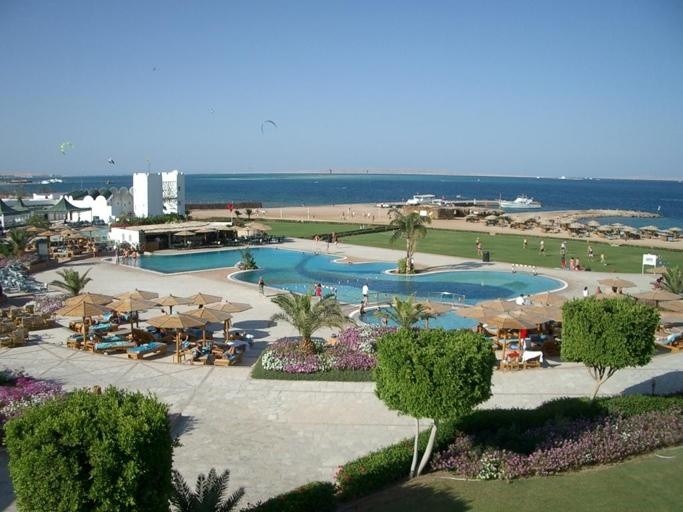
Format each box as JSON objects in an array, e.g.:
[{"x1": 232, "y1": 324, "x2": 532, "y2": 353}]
[{"x1": 67, "y1": 312, "x2": 254, "y2": 367}]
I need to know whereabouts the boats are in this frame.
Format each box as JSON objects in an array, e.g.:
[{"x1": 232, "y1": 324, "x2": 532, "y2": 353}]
[
  {"x1": 11, "y1": 178, "x2": 33, "y2": 183},
  {"x1": 41, "y1": 177, "x2": 64, "y2": 185},
  {"x1": 499, "y1": 193, "x2": 543, "y2": 208},
  {"x1": 407, "y1": 194, "x2": 438, "y2": 206}
]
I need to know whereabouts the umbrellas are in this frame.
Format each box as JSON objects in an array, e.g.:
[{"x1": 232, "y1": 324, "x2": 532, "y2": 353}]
[
  {"x1": 409, "y1": 265, "x2": 682, "y2": 373},
  {"x1": 172, "y1": 220, "x2": 271, "y2": 249},
  {"x1": 464, "y1": 211, "x2": 682, "y2": 243},
  {"x1": 16, "y1": 223, "x2": 101, "y2": 256},
  {"x1": 52, "y1": 288, "x2": 253, "y2": 365}
]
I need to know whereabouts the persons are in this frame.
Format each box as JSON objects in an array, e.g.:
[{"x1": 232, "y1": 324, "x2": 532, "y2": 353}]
[
  {"x1": 315, "y1": 284, "x2": 321, "y2": 297},
  {"x1": 359, "y1": 301, "x2": 365, "y2": 317},
  {"x1": 257, "y1": 276, "x2": 266, "y2": 297},
  {"x1": 474, "y1": 236, "x2": 606, "y2": 274},
  {"x1": 112, "y1": 240, "x2": 145, "y2": 265},
  {"x1": 361, "y1": 282, "x2": 368, "y2": 307}
]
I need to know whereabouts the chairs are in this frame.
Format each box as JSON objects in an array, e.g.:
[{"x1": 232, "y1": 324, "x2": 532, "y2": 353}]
[
  {"x1": 483, "y1": 322, "x2": 563, "y2": 372},
  {"x1": 656, "y1": 331, "x2": 683, "y2": 351},
  {"x1": 0, "y1": 304, "x2": 55, "y2": 348}
]
